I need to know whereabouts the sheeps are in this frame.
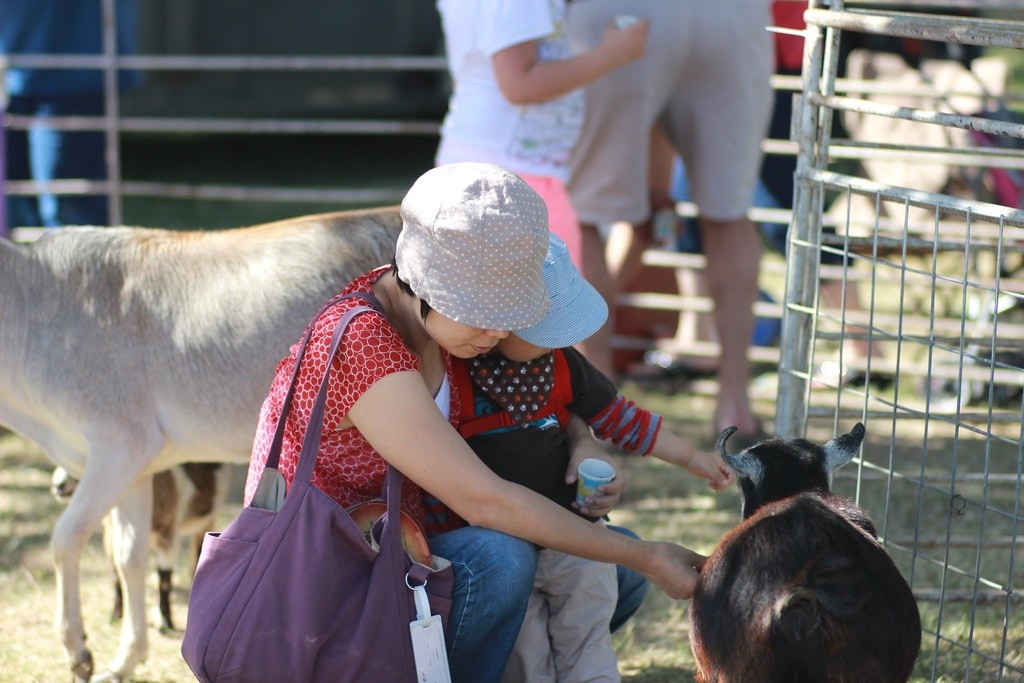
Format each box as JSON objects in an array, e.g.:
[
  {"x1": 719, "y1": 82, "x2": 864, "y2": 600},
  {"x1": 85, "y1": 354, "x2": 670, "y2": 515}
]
[
  {"x1": 687, "y1": 422, "x2": 920, "y2": 683},
  {"x1": 0, "y1": 204, "x2": 406, "y2": 683}
]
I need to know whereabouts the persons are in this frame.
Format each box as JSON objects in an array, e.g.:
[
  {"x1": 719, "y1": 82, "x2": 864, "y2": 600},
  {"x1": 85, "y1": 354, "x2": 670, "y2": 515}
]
[
  {"x1": 623, "y1": 0, "x2": 1023, "y2": 401},
  {"x1": 242, "y1": 163, "x2": 713, "y2": 683},
  {"x1": 0, "y1": 0, "x2": 151, "y2": 227},
  {"x1": 449, "y1": 230, "x2": 735, "y2": 682},
  {"x1": 435, "y1": 0, "x2": 777, "y2": 454}
]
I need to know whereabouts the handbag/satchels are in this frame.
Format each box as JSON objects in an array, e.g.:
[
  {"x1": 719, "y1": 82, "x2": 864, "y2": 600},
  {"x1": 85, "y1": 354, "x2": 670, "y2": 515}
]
[{"x1": 181, "y1": 292, "x2": 453, "y2": 683}]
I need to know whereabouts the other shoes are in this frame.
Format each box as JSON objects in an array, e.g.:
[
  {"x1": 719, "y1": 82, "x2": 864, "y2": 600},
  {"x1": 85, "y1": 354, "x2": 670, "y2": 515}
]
[{"x1": 712, "y1": 421, "x2": 772, "y2": 454}]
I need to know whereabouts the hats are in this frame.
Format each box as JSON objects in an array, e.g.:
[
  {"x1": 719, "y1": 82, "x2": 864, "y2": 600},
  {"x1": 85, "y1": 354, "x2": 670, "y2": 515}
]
[
  {"x1": 510, "y1": 230, "x2": 609, "y2": 349},
  {"x1": 396, "y1": 162, "x2": 552, "y2": 331}
]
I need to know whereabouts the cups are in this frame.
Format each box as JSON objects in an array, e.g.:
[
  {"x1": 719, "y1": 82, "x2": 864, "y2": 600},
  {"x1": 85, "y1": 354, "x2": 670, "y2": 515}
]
[{"x1": 576, "y1": 458, "x2": 616, "y2": 506}]
